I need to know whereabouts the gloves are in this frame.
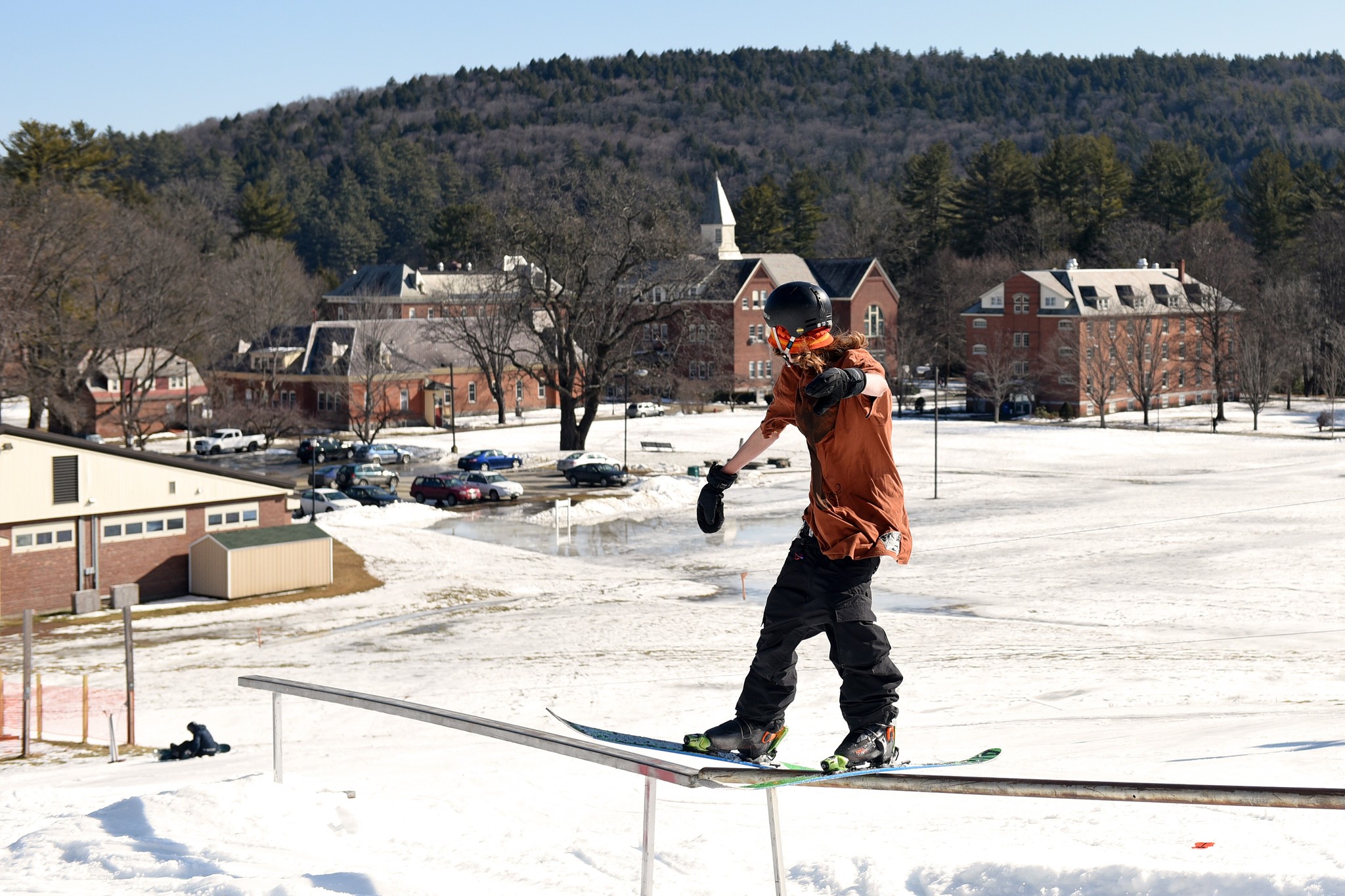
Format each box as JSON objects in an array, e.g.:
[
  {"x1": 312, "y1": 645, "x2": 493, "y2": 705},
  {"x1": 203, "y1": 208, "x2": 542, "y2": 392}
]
[
  {"x1": 805, "y1": 367, "x2": 866, "y2": 416},
  {"x1": 698, "y1": 460, "x2": 738, "y2": 533}
]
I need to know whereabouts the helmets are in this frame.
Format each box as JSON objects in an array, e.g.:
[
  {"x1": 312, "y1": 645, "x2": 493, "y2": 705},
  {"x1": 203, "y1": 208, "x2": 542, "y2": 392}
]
[{"x1": 763, "y1": 281, "x2": 833, "y2": 338}]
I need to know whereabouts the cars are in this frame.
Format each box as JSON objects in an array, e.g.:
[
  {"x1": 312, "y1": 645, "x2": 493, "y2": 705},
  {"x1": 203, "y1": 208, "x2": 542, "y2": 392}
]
[
  {"x1": 557, "y1": 450, "x2": 623, "y2": 477},
  {"x1": 293, "y1": 488, "x2": 362, "y2": 518},
  {"x1": 354, "y1": 443, "x2": 413, "y2": 466},
  {"x1": 458, "y1": 449, "x2": 523, "y2": 471},
  {"x1": 307, "y1": 465, "x2": 342, "y2": 489},
  {"x1": 565, "y1": 462, "x2": 629, "y2": 488},
  {"x1": 344, "y1": 484, "x2": 403, "y2": 507}
]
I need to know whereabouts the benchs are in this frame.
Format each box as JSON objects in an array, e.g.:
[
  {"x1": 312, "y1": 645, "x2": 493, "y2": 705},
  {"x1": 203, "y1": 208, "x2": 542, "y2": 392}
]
[{"x1": 641, "y1": 441, "x2": 676, "y2": 452}]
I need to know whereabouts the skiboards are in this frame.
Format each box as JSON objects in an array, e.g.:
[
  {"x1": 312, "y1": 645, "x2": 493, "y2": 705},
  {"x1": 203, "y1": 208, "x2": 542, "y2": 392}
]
[{"x1": 542, "y1": 704, "x2": 1001, "y2": 794}]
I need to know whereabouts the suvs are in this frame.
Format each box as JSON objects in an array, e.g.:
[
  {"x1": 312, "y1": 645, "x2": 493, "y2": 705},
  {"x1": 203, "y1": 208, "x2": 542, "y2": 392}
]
[
  {"x1": 458, "y1": 470, "x2": 524, "y2": 502},
  {"x1": 627, "y1": 401, "x2": 665, "y2": 418},
  {"x1": 409, "y1": 473, "x2": 483, "y2": 507},
  {"x1": 336, "y1": 462, "x2": 400, "y2": 489},
  {"x1": 297, "y1": 435, "x2": 357, "y2": 464}
]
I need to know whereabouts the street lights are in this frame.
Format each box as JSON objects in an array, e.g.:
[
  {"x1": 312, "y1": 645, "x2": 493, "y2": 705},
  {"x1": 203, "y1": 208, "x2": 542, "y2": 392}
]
[
  {"x1": 917, "y1": 365, "x2": 939, "y2": 499},
  {"x1": 620, "y1": 369, "x2": 648, "y2": 466},
  {"x1": 440, "y1": 362, "x2": 455, "y2": 445},
  {"x1": 177, "y1": 361, "x2": 191, "y2": 452}
]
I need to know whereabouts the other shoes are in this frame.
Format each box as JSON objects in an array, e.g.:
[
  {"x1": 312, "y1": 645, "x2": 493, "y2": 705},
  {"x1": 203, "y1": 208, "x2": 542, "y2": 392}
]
[
  {"x1": 835, "y1": 724, "x2": 895, "y2": 763},
  {"x1": 703, "y1": 714, "x2": 775, "y2": 758}
]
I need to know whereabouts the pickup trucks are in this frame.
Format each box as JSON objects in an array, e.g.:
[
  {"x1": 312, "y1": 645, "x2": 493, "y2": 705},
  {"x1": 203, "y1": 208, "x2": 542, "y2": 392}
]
[{"x1": 194, "y1": 428, "x2": 269, "y2": 456}]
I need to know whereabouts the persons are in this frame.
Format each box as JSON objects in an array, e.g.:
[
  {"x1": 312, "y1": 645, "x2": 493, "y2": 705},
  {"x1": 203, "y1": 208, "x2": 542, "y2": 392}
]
[
  {"x1": 683, "y1": 283, "x2": 913, "y2": 773},
  {"x1": 170, "y1": 722, "x2": 217, "y2": 758}
]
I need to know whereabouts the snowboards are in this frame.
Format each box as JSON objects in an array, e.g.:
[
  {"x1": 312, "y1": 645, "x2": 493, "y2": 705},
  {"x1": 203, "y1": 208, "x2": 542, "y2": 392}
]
[{"x1": 153, "y1": 744, "x2": 231, "y2": 760}]
